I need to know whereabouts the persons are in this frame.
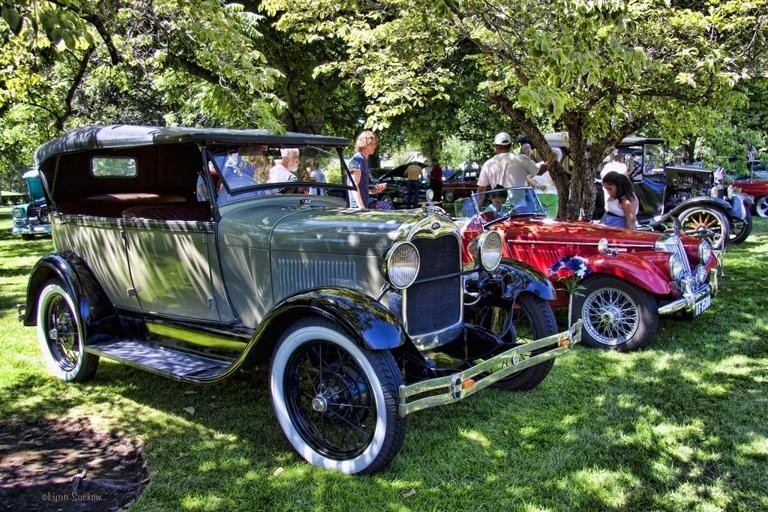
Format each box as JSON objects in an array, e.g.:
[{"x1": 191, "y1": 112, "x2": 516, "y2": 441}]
[
  {"x1": 197, "y1": 176, "x2": 207, "y2": 202},
  {"x1": 599, "y1": 149, "x2": 639, "y2": 230},
  {"x1": 404, "y1": 161, "x2": 423, "y2": 209},
  {"x1": 304, "y1": 162, "x2": 326, "y2": 196},
  {"x1": 269, "y1": 147, "x2": 300, "y2": 182},
  {"x1": 476, "y1": 132, "x2": 562, "y2": 219},
  {"x1": 346, "y1": 131, "x2": 387, "y2": 209},
  {"x1": 430, "y1": 159, "x2": 444, "y2": 208}
]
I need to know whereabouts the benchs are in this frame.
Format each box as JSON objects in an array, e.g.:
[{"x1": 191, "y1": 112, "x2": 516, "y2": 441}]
[
  {"x1": 59, "y1": 190, "x2": 188, "y2": 216},
  {"x1": 123, "y1": 201, "x2": 212, "y2": 222}
]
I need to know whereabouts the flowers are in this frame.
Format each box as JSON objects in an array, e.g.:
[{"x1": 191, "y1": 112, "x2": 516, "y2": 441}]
[{"x1": 547, "y1": 244, "x2": 593, "y2": 329}]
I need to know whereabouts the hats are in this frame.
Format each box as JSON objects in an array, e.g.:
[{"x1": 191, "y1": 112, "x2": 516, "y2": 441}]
[{"x1": 494, "y1": 133, "x2": 511, "y2": 145}]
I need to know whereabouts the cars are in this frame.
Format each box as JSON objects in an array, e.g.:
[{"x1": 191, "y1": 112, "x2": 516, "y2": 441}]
[
  {"x1": 591, "y1": 138, "x2": 768, "y2": 250},
  {"x1": 17, "y1": 124, "x2": 586, "y2": 476},
  {"x1": 12, "y1": 169, "x2": 52, "y2": 241},
  {"x1": 440, "y1": 168, "x2": 492, "y2": 204},
  {"x1": 366, "y1": 161, "x2": 434, "y2": 210},
  {"x1": 459, "y1": 185, "x2": 721, "y2": 352}
]
